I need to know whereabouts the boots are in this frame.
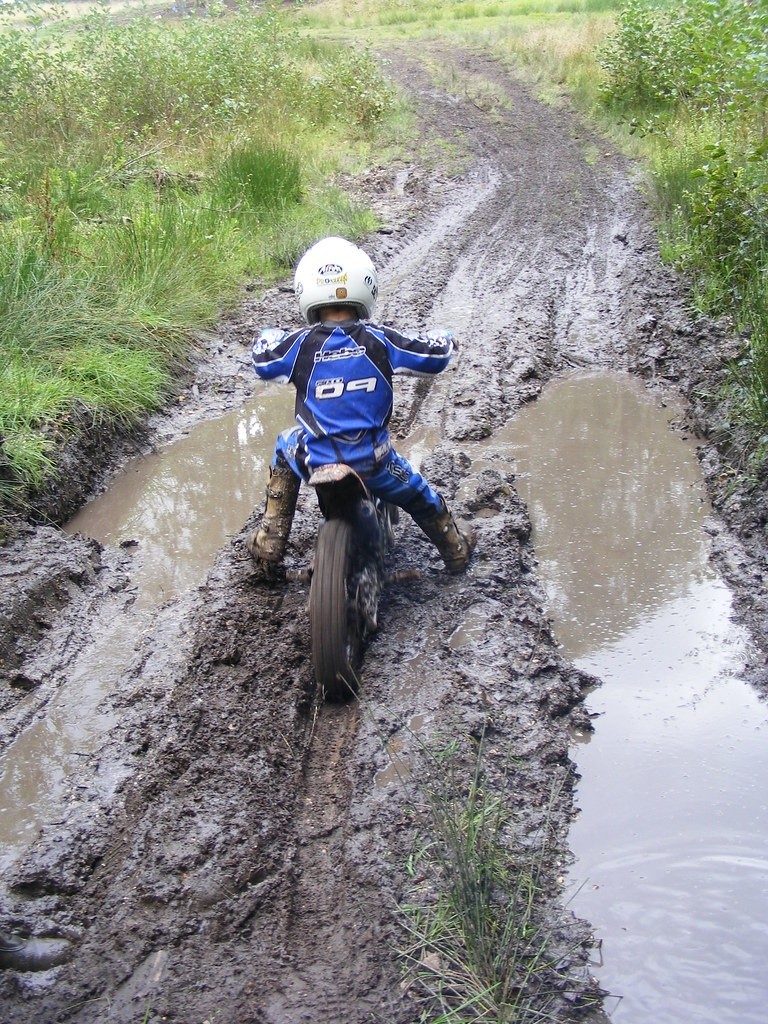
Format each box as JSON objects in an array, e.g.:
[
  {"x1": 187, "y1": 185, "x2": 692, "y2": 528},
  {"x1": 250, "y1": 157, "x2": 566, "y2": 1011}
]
[
  {"x1": 412, "y1": 493, "x2": 476, "y2": 570},
  {"x1": 246, "y1": 463, "x2": 303, "y2": 562}
]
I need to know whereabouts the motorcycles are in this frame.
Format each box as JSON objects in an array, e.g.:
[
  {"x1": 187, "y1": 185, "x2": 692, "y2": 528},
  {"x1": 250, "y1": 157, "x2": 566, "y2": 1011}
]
[{"x1": 268, "y1": 331, "x2": 460, "y2": 692}]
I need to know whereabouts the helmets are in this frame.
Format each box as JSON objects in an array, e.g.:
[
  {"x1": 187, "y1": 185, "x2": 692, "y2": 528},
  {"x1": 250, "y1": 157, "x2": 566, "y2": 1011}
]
[{"x1": 294, "y1": 236, "x2": 380, "y2": 324}]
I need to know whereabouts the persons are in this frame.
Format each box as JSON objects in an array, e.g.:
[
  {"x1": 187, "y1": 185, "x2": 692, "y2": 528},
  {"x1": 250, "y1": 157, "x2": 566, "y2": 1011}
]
[{"x1": 248, "y1": 239, "x2": 476, "y2": 573}]
